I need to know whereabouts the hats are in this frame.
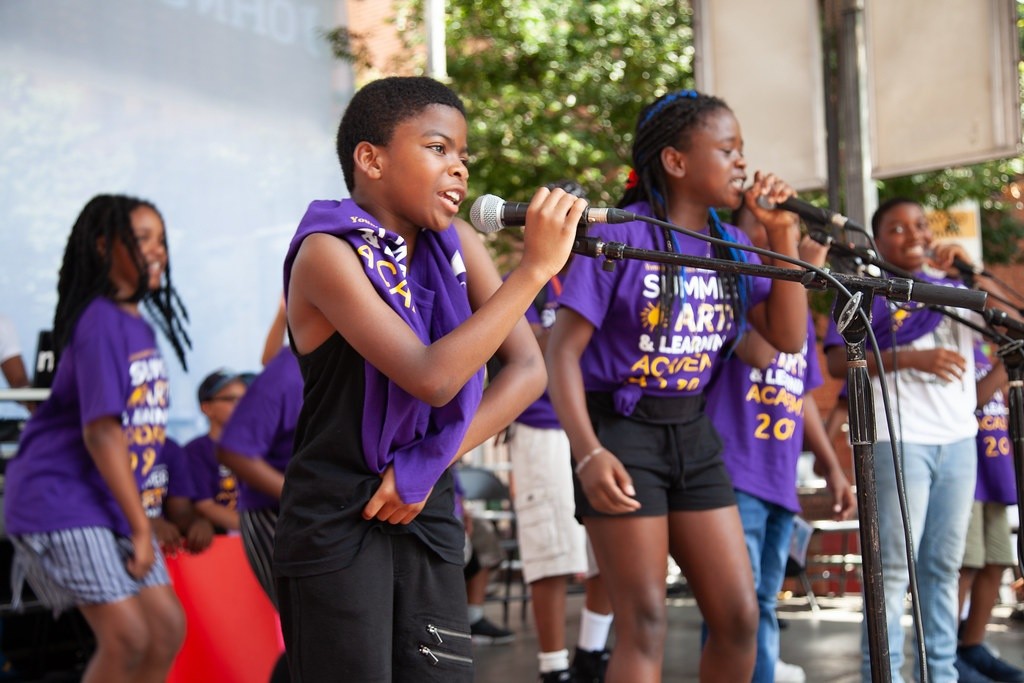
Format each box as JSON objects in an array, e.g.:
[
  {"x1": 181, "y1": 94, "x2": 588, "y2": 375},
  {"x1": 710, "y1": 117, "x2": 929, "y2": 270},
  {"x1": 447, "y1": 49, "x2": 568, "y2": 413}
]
[{"x1": 198, "y1": 370, "x2": 258, "y2": 400}]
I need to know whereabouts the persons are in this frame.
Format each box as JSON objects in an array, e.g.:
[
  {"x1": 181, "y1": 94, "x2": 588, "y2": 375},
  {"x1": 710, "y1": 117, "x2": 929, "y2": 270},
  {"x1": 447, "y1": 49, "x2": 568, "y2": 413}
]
[
  {"x1": 155, "y1": 191, "x2": 1024, "y2": 683},
  {"x1": 822, "y1": 200, "x2": 979, "y2": 683},
  {"x1": 1, "y1": 193, "x2": 192, "y2": 683},
  {"x1": 546, "y1": 88, "x2": 808, "y2": 683},
  {"x1": 271, "y1": 74, "x2": 587, "y2": 683}
]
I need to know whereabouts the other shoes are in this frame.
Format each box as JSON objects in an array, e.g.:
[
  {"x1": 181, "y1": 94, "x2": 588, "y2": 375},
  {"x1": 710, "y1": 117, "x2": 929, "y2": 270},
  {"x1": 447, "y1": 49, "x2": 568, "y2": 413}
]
[
  {"x1": 954, "y1": 645, "x2": 1024, "y2": 683},
  {"x1": 773, "y1": 660, "x2": 805, "y2": 683},
  {"x1": 540, "y1": 646, "x2": 612, "y2": 683}
]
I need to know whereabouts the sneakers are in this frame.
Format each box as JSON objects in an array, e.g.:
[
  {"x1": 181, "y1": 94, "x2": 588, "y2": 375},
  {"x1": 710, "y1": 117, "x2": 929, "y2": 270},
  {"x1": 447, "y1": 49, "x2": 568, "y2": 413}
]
[{"x1": 471, "y1": 617, "x2": 516, "y2": 646}]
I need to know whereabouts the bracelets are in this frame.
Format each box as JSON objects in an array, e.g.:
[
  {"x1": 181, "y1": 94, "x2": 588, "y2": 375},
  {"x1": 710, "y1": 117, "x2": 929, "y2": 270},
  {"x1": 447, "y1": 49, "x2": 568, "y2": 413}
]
[{"x1": 574, "y1": 448, "x2": 608, "y2": 476}]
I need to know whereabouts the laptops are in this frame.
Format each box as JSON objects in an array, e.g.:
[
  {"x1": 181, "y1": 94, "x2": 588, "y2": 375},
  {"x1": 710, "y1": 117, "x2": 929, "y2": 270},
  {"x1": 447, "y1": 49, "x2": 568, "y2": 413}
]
[{"x1": 16, "y1": 327, "x2": 58, "y2": 388}]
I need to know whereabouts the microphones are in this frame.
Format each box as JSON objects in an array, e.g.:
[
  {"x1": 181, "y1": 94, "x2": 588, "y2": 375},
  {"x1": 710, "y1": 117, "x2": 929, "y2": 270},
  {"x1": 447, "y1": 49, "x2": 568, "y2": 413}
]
[
  {"x1": 469, "y1": 194, "x2": 636, "y2": 232},
  {"x1": 827, "y1": 248, "x2": 869, "y2": 258},
  {"x1": 757, "y1": 193, "x2": 863, "y2": 233},
  {"x1": 922, "y1": 250, "x2": 991, "y2": 277}
]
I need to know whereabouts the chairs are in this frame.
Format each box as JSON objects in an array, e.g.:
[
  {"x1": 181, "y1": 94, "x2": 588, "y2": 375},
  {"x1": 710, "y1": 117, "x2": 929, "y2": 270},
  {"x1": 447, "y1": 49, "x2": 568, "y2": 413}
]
[{"x1": 455, "y1": 466, "x2": 530, "y2": 626}]
